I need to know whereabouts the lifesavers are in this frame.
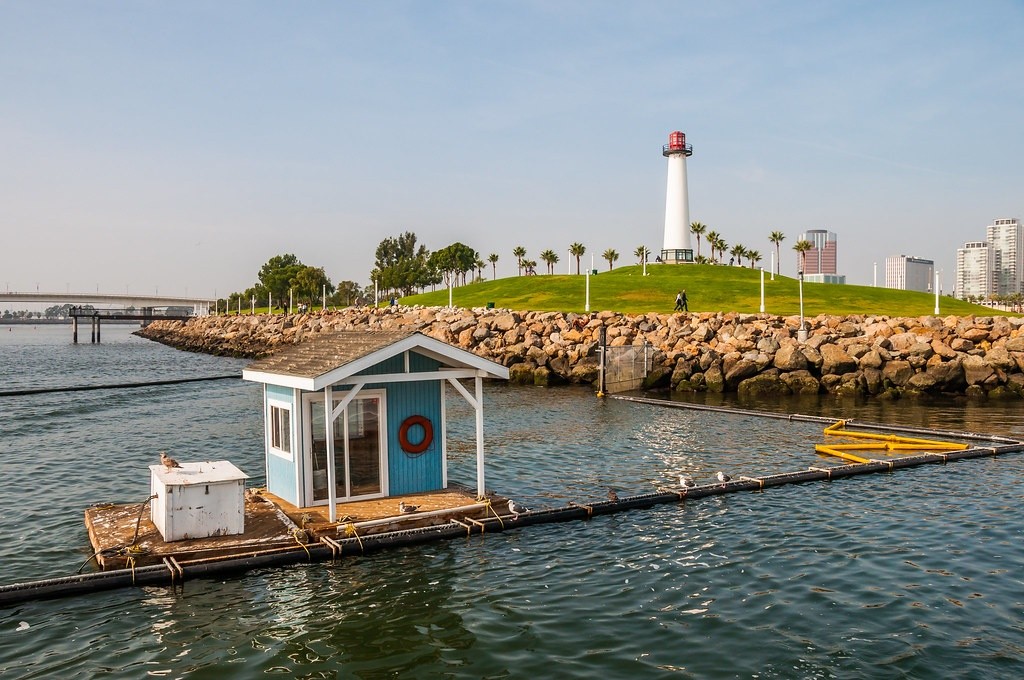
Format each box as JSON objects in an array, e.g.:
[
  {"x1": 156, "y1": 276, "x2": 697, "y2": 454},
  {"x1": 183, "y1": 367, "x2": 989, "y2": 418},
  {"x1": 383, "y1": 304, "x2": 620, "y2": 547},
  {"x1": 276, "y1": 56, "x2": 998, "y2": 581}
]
[{"x1": 398, "y1": 414, "x2": 435, "y2": 455}]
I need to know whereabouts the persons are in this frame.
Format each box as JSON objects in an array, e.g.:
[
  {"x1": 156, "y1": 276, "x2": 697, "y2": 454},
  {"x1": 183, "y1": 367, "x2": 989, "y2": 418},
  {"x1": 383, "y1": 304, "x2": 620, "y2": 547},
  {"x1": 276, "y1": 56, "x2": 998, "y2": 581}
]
[
  {"x1": 355, "y1": 297, "x2": 359, "y2": 309},
  {"x1": 217, "y1": 307, "x2": 226, "y2": 315},
  {"x1": 72, "y1": 305, "x2": 82, "y2": 315},
  {"x1": 390, "y1": 297, "x2": 398, "y2": 308},
  {"x1": 326, "y1": 304, "x2": 337, "y2": 311},
  {"x1": 674, "y1": 289, "x2": 690, "y2": 312},
  {"x1": 284, "y1": 302, "x2": 287, "y2": 317},
  {"x1": 297, "y1": 301, "x2": 307, "y2": 314},
  {"x1": 729, "y1": 257, "x2": 734, "y2": 266},
  {"x1": 655, "y1": 256, "x2": 661, "y2": 262}
]
